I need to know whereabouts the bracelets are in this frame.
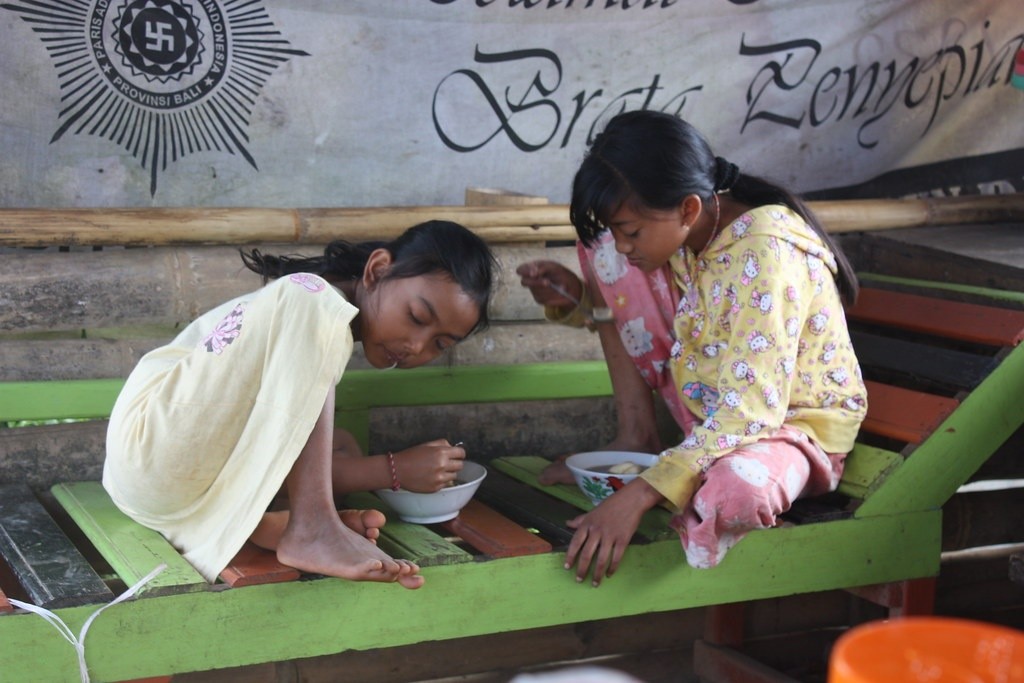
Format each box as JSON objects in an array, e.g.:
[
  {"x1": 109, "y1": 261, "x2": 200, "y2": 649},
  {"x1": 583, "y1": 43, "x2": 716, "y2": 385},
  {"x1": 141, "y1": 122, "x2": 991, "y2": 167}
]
[{"x1": 388, "y1": 452, "x2": 401, "y2": 493}]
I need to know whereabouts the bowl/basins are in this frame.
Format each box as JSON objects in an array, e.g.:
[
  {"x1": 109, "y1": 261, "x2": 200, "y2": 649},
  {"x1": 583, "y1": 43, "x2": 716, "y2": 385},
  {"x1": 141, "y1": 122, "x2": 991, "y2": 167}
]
[
  {"x1": 376, "y1": 461, "x2": 487, "y2": 523},
  {"x1": 565, "y1": 451, "x2": 660, "y2": 507}
]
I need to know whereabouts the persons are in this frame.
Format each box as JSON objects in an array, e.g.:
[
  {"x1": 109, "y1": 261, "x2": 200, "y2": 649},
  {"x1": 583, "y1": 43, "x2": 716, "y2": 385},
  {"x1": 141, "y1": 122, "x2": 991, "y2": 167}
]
[
  {"x1": 516, "y1": 109, "x2": 869, "y2": 587},
  {"x1": 100, "y1": 219, "x2": 491, "y2": 588}
]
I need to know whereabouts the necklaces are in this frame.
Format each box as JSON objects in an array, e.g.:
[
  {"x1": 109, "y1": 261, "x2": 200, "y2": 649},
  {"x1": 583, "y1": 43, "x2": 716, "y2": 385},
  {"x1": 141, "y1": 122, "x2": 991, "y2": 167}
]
[{"x1": 680, "y1": 191, "x2": 721, "y2": 287}]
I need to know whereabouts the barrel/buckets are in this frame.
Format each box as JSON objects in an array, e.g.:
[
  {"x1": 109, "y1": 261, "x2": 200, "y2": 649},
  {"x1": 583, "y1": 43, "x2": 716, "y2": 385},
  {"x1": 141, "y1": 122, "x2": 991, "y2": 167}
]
[{"x1": 828, "y1": 617, "x2": 1024, "y2": 683}]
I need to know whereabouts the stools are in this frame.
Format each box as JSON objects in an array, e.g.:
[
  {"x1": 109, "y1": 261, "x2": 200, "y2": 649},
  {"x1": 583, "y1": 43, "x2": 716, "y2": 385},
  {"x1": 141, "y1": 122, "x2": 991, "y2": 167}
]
[{"x1": 829, "y1": 617, "x2": 1024, "y2": 683}]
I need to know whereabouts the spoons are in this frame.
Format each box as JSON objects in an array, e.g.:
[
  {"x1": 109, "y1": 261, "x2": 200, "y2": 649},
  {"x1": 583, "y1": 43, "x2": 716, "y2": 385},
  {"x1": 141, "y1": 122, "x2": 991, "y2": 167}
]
[{"x1": 544, "y1": 278, "x2": 618, "y2": 325}]
